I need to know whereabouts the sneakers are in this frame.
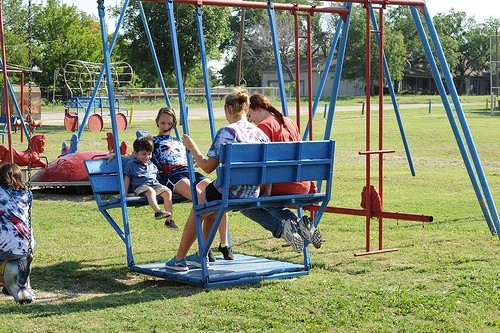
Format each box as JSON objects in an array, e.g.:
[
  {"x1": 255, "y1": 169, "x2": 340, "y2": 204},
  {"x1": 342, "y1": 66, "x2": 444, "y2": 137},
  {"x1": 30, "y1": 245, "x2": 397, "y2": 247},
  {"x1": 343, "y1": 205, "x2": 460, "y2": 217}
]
[
  {"x1": 186, "y1": 252, "x2": 209, "y2": 268},
  {"x1": 209, "y1": 250, "x2": 216, "y2": 262},
  {"x1": 297, "y1": 215, "x2": 322, "y2": 248},
  {"x1": 218, "y1": 242, "x2": 233, "y2": 260},
  {"x1": 281, "y1": 219, "x2": 303, "y2": 254},
  {"x1": 165, "y1": 256, "x2": 189, "y2": 270}
]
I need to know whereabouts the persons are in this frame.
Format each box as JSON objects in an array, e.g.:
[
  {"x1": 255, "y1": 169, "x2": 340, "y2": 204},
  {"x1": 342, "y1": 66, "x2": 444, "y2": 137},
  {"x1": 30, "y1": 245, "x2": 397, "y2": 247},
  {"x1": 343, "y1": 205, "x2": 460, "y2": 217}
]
[
  {"x1": 166, "y1": 90, "x2": 272, "y2": 272},
  {"x1": 152, "y1": 107, "x2": 235, "y2": 262},
  {"x1": 124, "y1": 138, "x2": 178, "y2": 231},
  {"x1": 246, "y1": 94, "x2": 322, "y2": 254},
  {"x1": 0, "y1": 164, "x2": 34, "y2": 295}
]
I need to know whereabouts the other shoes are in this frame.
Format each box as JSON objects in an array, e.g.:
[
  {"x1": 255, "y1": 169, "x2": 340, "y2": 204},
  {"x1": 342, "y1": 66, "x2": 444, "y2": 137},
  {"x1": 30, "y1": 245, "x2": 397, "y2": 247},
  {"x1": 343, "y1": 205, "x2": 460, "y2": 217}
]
[
  {"x1": 155, "y1": 209, "x2": 171, "y2": 219},
  {"x1": 164, "y1": 220, "x2": 178, "y2": 230}
]
